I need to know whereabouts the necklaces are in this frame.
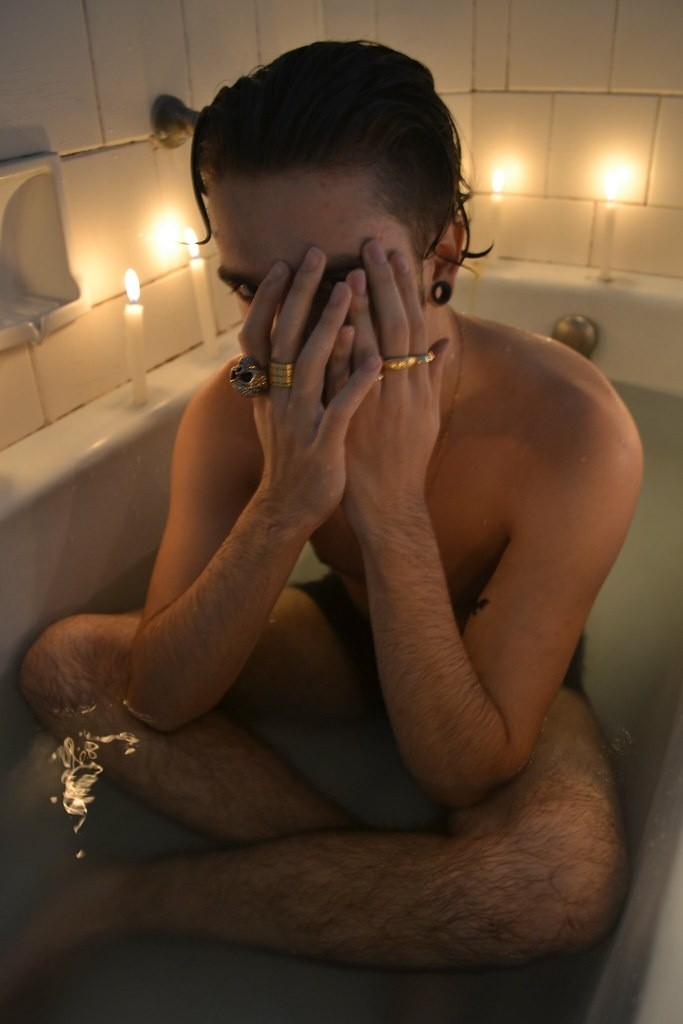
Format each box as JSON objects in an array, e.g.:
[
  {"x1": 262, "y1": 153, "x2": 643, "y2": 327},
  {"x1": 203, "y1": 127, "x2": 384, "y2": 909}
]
[{"x1": 426, "y1": 305, "x2": 462, "y2": 501}]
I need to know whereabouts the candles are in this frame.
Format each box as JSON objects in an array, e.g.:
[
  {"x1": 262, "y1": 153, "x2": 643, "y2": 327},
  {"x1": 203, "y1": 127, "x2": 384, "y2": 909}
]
[
  {"x1": 597, "y1": 198, "x2": 618, "y2": 281},
  {"x1": 189, "y1": 252, "x2": 219, "y2": 359},
  {"x1": 123, "y1": 297, "x2": 147, "y2": 404},
  {"x1": 488, "y1": 191, "x2": 501, "y2": 267}
]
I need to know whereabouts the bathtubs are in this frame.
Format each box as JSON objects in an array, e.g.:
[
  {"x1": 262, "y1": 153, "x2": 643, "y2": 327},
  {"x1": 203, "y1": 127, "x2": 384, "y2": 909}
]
[{"x1": 1, "y1": 246, "x2": 681, "y2": 1024}]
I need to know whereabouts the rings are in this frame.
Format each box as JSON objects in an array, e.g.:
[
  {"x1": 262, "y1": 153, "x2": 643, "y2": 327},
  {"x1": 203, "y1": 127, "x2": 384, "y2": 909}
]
[
  {"x1": 266, "y1": 358, "x2": 294, "y2": 387},
  {"x1": 228, "y1": 356, "x2": 271, "y2": 398},
  {"x1": 413, "y1": 351, "x2": 436, "y2": 365},
  {"x1": 382, "y1": 354, "x2": 416, "y2": 372},
  {"x1": 375, "y1": 373, "x2": 385, "y2": 382}
]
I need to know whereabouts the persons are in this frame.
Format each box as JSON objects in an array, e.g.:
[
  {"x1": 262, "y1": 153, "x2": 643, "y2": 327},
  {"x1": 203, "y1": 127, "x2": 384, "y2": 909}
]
[{"x1": 0, "y1": 40, "x2": 642, "y2": 996}]
